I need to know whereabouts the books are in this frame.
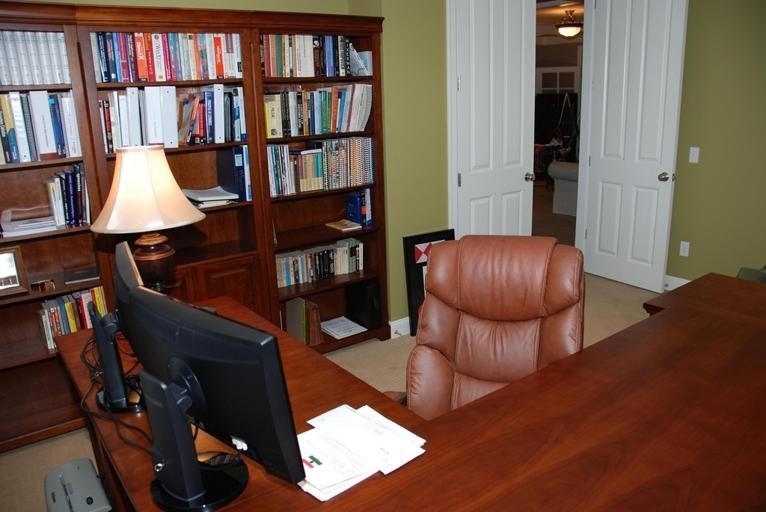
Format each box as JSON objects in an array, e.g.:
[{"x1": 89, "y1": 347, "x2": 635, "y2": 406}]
[
  {"x1": 1, "y1": 29, "x2": 71, "y2": 85},
  {"x1": 265, "y1": 83, "x2": 376, "y2": 138},
  {"x1": 0, "y1": 163, "x2": 92, "y2": 236},
  {"x1": 268, "y1": 140, "x2": 378, "y2": 196},
  {"x1": 65, "y1": 263, "x2": 100, "y2": 283},
  {"x1": 89, "y1": 30, "x2": 242, "y2": 82},
  {"x1": 276, "y1": 238, "x2": 364, "y2": 286},
  {"x1": 327, "y1": 218, "x2": 363, "y2": 233},
  {"x1": 287, "y1": 298, "x2": 322, "y2": 347},
  {"x1": 96, "y1": 84, "x2": 248, "y2": 152},
  {"x1": 260, "y1": 32, "x2": 374, "y2": 77},
  {"x1": 344, "y1": 188, "x2": 372, "y2": 225},
  {"x1": 40, "y1": 287, "x2": 107, "y2": 352},
  {"x1": 0, "y1": 88, "x2": 82, "y2": 163},
  {"x1": 183, "y1": 146, "x2": 252, "y2": 207}
]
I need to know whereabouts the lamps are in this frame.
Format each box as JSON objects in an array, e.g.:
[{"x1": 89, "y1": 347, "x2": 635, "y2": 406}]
[
  {"x1": 555, "y1": 10, "x2": 583, "y2": 39},
  {"x1": 91, "y1": 145, "x2": 217, "y2": 292}
]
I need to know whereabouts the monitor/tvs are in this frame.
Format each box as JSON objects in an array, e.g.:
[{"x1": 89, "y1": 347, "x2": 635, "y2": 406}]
[
  {"x1": 86, "y1": 241, "x2": 145, "y2": 414},
  {"x1": 128, "y1": 286, "x2": 305, "y2": 511}
]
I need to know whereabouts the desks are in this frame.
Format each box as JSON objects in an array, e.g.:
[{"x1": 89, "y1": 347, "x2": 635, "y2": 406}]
[{"x1": 54, "y1": 272, "x2": 766, "y2": 512}]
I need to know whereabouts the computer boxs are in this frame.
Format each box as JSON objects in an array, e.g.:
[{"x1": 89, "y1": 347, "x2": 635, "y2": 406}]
[{"x1": 44, "y1": 458, "x2": 112, "y2": 512}]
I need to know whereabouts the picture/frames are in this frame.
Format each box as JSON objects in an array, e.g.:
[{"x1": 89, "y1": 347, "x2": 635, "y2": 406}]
[
  {"x1": 0, "y1": 247, "x2": 31, "y2": 298},
  {"x1": 403, "y1": 229, "x2": 455, "y2": 336}
]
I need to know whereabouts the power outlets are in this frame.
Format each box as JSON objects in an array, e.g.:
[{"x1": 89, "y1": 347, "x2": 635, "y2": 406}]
[{"x1": 390, "y1": 317, "x2": 411, "y2": 338}]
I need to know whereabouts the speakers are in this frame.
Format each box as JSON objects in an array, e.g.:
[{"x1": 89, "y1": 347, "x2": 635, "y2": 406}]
[{"x1": 345, "y1": 279, "x2": 382, "y2": 329}]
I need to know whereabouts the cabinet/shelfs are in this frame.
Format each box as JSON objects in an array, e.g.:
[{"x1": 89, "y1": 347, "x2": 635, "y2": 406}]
[
  {"x1": 252, "y1": 10, "x2": 390, "y2": 354},
  {"x1": 0, "y1": 0, "x2": 117, "y2": 453},
  {"x1": 75, "y1": 0, "x2": 274, "y2": 327}
]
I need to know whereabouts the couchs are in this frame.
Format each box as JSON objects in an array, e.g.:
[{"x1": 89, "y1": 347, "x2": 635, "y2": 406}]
[
  {"x1": 547, "y1": 161, "x2": 578, "y2": 217},
  {"x1": 401, "y1": 235, "x2": 584, "y2": 420}
]
